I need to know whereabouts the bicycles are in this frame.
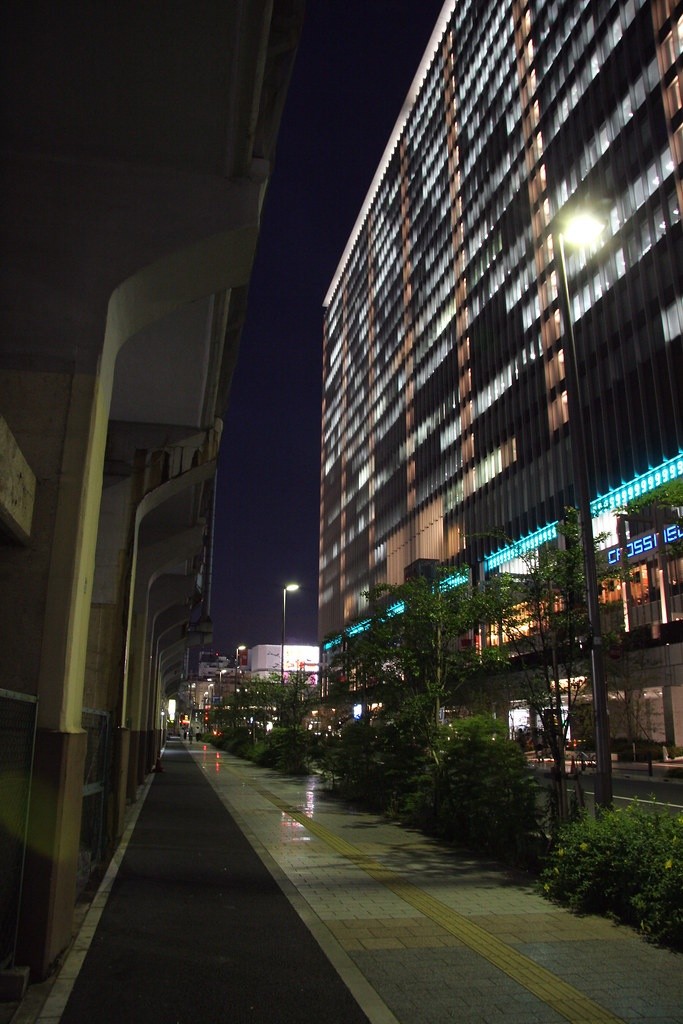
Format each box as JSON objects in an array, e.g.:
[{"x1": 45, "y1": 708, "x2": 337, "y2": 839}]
[{"x1": 576, "y1": 745, "x2": 597, "y2": 766}]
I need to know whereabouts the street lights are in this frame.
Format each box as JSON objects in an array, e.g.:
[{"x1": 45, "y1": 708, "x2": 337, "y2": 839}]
[
  {"x1": 280, "y1": 582, "x2": 301, "y2": 685},
  {"x1": 553, "y1": 194, "x2": 613, "y2": 820},
  {"x1": 234, "y1": 645, "x2": 246, "y2": 693}
]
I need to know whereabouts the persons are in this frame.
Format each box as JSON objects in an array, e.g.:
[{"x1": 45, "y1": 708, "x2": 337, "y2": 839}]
[
  {"x1": 189, "y1": 727, "x2": 193, "y2": 744},
  {"x1": 535, "y1": 733, "x2": 544, "y2": 762},
  {"x1": 516, "y1": 728, "x2": 526, "y2": 754},
  {"x1": 195, "y1": 727, "x2": 201, "y2": 742}
]
